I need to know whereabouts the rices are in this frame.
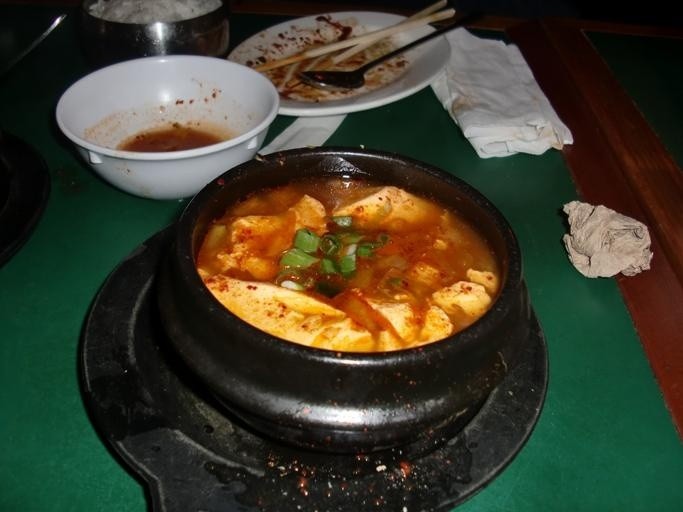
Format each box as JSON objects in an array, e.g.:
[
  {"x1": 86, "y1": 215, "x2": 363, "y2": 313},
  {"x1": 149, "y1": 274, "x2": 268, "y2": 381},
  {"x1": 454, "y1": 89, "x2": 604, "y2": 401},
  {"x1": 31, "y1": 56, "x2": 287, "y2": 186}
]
[{"x1": 89, "y1": 0, "x2": 222, "y2": 24}]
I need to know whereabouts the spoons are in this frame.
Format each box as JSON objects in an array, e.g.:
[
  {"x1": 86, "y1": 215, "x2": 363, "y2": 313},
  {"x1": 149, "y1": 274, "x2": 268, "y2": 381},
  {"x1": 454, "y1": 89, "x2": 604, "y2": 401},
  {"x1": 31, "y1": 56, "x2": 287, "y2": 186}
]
[{"x1": 294, "y1": 11, "x2": 486, "y2": 92}]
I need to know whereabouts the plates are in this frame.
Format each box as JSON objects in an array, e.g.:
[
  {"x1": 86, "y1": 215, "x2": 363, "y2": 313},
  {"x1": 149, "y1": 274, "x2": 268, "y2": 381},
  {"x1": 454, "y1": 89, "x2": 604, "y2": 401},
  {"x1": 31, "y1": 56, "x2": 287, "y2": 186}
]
[
  {"x1": 77, "y1": 216, "x2": 551, "y2": 512},
  {"x1": 226, "y1": 10, "x2": 451, "y2": 117}
]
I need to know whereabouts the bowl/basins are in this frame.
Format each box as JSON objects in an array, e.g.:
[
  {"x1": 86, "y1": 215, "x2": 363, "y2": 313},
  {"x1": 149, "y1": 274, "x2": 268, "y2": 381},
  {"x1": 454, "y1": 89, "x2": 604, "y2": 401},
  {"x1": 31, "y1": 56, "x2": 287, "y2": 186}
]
[
  {"x1": 157, "y1": 145, "x2": 522, "y2": 458},
  {"x1": 81, "y1": 1, "x2": 231, "y2": 67},
  {"x1": 54, "y1": 54, "x2": 281, "y2": 201}
]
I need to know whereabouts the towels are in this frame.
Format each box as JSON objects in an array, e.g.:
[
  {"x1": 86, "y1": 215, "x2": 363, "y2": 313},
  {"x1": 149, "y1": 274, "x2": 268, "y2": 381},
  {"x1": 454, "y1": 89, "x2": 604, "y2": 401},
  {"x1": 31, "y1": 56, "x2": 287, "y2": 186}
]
[{"x1": 430, "y1": 26, "x2": 575, "y2": 159}]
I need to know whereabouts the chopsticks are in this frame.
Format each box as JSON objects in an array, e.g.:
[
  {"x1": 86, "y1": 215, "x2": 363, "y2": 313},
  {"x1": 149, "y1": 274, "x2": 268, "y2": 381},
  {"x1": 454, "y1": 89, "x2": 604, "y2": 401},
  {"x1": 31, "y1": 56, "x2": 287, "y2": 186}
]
[{"x1": 249, "y1": 0, "x2": 457, "y2": 88}]
